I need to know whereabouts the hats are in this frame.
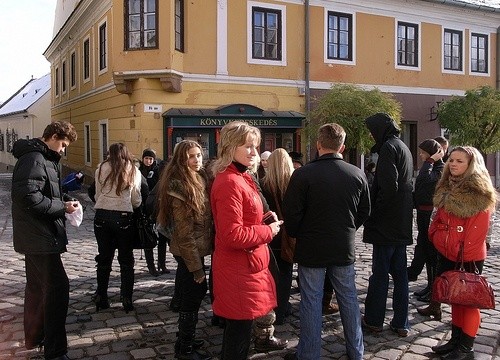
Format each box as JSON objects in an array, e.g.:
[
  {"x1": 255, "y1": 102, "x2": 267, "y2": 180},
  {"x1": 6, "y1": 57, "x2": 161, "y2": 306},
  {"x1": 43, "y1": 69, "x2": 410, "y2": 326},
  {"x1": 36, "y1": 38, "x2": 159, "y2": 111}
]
[
  {"x1": 289, "y1": 151, "x2": 304, "y2": 166},
  {"x1": 419, "y1": 139, "x2": 442, "y2": 156},
  {"x1": 142, "y1": 148, "x2": 156, "y2": 159},
  {"x1": 261, "y1": 151, "x2": 272, "y2": 160}
]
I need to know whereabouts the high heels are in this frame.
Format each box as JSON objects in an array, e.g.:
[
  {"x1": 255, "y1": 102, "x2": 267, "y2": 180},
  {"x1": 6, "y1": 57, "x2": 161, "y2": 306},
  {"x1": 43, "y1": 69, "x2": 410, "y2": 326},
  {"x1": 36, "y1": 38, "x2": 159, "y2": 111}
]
[{"x1": 417, "y1": 305, "x2": 442, "y2": 320}]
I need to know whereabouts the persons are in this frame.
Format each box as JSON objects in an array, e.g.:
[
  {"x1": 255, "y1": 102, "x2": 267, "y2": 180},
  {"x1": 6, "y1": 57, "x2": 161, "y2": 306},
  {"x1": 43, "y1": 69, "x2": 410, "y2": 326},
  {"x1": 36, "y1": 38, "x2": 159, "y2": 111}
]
[
  {"x1": 360, "y1": 112, "x2": 414, "y2": 336},
  {"x1": 136, "y1": 148, "x2": 170, "y2": 277},
  {"x1": 428, "y1": 146, "x2": 496, "y2": 360},
  {"x1": 10, "y1": 121, "x2": 79, "y2": 360},
  {"x1": 282, "y1": 123, "x2": 371, "y2": 360},
  {"x1": 157, "y1": 140, "x2": 214, "y2": 360},
  {"x1": 407, "y1": 135, "x2": 448, "y2": 281},
  {"x1": 413, "y1": 138, "x2": 445, "y2": 302},
  {"x1": 93, "y1": 143, "x2": 141, "y2": 313},
  {"x1": 252, "y1": 148, "x2": 339, "y2": 350},
  {"x1": 210, "y1": 121, "x2": 284, "y2": 360},
  {"x1": 365, "y1": 163, "x2": 376, "y2": 187}
]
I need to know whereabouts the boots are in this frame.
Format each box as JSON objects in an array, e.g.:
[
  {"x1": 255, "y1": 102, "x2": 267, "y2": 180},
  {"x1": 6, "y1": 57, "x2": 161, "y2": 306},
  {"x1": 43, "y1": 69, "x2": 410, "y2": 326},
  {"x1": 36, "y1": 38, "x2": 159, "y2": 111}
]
[
  {"x1": 431, "y1": 323, "x2": 462, "y2": 354},
  {"x1": 157, "y1": 245, "x2": 169, "y2": 273},
  {"x1": 121, "y1": 269, "x2": 134, "y2": 314},
  {"x1": 322, "y1": 293, "x2": 338, "y2": 313},
  {"x1": 94, "y1": 269, "x2": 110, "y2": 312},
  {"x1": 144, "y1": 249, "x2": 160, "y2": 277},
  {"x1": 253, "y1": 312, "x2": 288, "y2": 352},
  {"x1": 174, "y1": 312, "x2": 210, "y2": 359},
  {"x1": 439, "y1": 332, "x2": 476, "y2": 360}
]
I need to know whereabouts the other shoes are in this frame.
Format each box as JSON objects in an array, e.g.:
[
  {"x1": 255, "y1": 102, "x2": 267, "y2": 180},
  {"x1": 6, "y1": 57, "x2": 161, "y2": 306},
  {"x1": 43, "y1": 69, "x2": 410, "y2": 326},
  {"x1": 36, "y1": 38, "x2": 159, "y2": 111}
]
[
  {"x1": 417, "y1": 291, "x2": 431, "y2": 302},
  {"x1": 362, "y1": 316, "x2": 383, "y2": 331},
  {"x1": 414, "y1": 285, "x2": 430, "y2": 295},
  {"x1": 390, "y1": 321, "x2": 409, "y2": 336}
]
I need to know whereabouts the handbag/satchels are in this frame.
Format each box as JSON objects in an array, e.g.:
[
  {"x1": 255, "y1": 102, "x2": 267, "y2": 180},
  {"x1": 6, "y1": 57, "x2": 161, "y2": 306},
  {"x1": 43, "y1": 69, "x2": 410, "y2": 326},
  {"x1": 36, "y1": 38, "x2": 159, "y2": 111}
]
[
  {"x1": 281, "y1": 230, "x2": 297, "y2": 264},
  {"x1": 431, "y1": 242, "x2": 496, "y2": 310},
  {"x1": 131, "y1": 206, "x2": 157, "y2": 249}
]
